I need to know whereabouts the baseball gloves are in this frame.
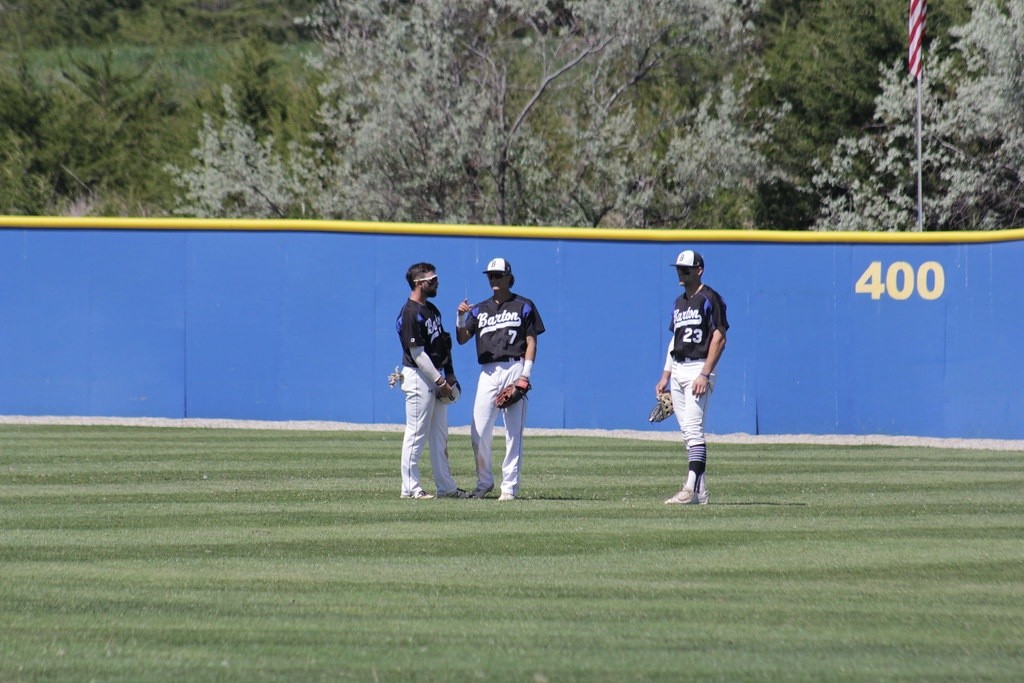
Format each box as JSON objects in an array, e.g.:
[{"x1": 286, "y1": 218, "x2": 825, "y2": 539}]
[
  {"x1": 495, "y1": 375, "x2": 532, "y2": 409},
  {"x1": 647, "y1": 389, "x2": 674, "y2": 424}
]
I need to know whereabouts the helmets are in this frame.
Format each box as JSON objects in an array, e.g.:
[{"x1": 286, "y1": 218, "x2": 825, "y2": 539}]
[{"x1": 436, "y1": 381, "x2": 461, "y2": 403}]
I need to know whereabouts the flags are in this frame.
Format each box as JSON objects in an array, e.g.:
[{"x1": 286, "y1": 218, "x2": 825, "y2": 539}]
[{"x1": 909, "y1": 0, "x2": 927, "y2": 81}]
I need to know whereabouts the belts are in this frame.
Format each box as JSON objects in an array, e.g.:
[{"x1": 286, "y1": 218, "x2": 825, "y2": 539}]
[
  {"x1": 675, "y1": 356, "x2": 698, "y2": 362},
  {"x1": 493, "y1": 356, "x2": 520, "y2": 362}
]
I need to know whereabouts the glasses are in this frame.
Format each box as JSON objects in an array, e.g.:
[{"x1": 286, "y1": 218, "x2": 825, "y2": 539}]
[
  {"x1": 414, "y1": 276, "x2": 438, "y2": 284},
  {"x1": 675, "y1": 266, "x2": 697, "y2": 270},
  {"x1": 487, "y1": 273, "x2": 509, "y2": 280}
]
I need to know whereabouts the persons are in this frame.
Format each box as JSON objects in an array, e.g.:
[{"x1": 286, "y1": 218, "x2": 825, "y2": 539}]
[
  {"x1": 456, "y1": 258, "x2": 545, "y2": 500},
  {"x1": 396, "y1": 262, "x2": 475, "y2": 499},
  {"x1": 656, "y1": 250, "x2": 730, "y2": 503}
]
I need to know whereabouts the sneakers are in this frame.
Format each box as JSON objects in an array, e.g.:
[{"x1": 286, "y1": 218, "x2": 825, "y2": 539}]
[
  {"x1": 665, "y1": 488, "x2": 698, "y2": 505},
  {"x1": 436, "y1": 487, "x2": 470, "y2": 498},
  {"x1": 697, "y1": 490, "x2": 709, "y2": 505},
  {"x1": 471, "y1": 483, "x2": 494, "y2": 498},
  {"x1": 499, "y1": 493, "x2": 514, "y2": 501},
  {"x1": 401, "y1": 490, "x2": 435, "y2": 499}
]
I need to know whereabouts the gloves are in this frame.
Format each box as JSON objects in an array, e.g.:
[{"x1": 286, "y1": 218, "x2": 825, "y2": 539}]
[{"x1": 388, "y1": 366, "x2": 403, "y2": 387}]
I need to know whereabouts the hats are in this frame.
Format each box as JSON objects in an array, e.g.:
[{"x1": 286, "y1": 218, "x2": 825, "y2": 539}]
[
  {"x1": 670, "y1": 250, "x2": 704, "y2": 268},
  {"x1": 483, "y1": 258, "x2": 512, "y2": 273}
]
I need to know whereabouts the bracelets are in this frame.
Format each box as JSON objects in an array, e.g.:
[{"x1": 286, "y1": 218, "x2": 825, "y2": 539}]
[{"x1": 700, "y1": 373, "x2": 709, "y2": 378}]
